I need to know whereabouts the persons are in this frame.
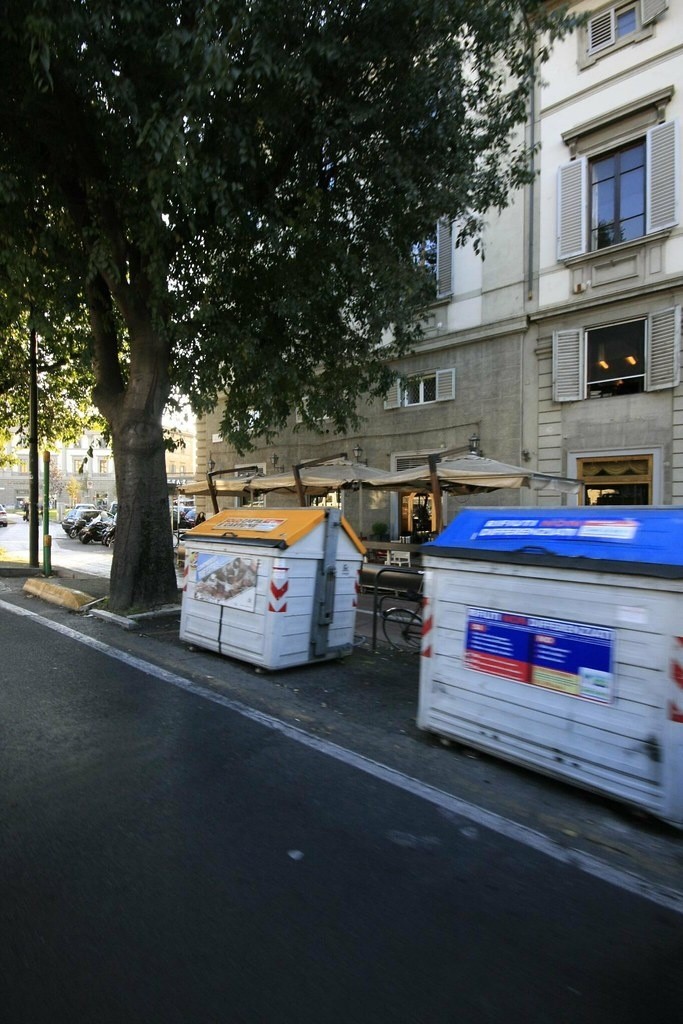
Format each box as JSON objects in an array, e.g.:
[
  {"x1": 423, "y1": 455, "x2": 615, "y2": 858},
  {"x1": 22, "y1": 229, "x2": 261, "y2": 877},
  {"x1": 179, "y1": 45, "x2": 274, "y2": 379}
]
[
  {"x1": 195, "y1": 511, "x2": 205, "y2": 525},
  {"x1": 23, "y1": 502, "x2": 29, "y2": 521}
]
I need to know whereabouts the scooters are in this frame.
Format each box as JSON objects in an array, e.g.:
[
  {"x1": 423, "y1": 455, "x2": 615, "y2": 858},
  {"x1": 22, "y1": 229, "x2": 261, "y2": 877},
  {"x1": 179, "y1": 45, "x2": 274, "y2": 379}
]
[{"x1": 69, "y1": 517, "x2": 116, "y2": 547}]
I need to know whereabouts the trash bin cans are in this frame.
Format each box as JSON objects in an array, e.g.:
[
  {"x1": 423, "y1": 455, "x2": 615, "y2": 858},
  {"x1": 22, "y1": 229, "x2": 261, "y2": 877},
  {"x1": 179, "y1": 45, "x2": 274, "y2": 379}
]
[
  {"x1": 415, "y1": 505, "x2": 682, "y2": 833},
  {"x1": 177, "y1": 506, "x2": 367, "y2": 672}
]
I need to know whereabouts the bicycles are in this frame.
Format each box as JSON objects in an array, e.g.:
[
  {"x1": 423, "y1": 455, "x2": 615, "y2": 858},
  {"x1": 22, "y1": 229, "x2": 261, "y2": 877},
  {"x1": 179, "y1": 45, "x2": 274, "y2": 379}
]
[{"x1": 383, "y1": 597, "x2": 423, "y2": 655}]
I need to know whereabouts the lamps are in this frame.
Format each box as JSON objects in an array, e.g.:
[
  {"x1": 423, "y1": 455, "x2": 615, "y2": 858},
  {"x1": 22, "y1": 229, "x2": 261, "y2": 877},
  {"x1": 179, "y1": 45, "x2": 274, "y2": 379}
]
[
  {"x1": 270, "y1": 452, "x2": 284, "y2": 472},
  {"x1": 469, "y1": 431, "x2": 483, "y2": 458},
  {"x1": 208, "y1": 457, "x2": 221, "y2": 479},
  {"x1": 354, "y1": 444, "x2": 367, "y2": 466}
]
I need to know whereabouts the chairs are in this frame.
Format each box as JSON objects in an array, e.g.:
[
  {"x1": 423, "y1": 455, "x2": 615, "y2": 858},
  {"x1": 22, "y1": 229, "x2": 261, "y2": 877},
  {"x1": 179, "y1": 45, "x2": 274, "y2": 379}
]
[{"x1": 386, "y1": 535, "x2": 412, "y2": 566}]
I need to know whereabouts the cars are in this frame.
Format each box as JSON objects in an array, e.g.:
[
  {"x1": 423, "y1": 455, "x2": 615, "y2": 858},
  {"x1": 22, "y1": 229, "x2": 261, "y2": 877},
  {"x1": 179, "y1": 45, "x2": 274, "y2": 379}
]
[
  {"x1": 0, "y1": 504, "x2": 7, "y2": 527},
  {"x1": 75, "y1": 503, "x2": 97, "y2": 510},
  {"x1": 109, "y1": 501, "x2": 196, "y2": 530},
  {"x1": 62, "y1": 510, "x2": 115, "y2": 535}
]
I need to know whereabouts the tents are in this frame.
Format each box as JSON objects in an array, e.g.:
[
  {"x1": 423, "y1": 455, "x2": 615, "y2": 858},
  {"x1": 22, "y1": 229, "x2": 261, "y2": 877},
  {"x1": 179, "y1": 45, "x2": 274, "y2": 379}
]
[{"x1": 178, "y1": 457, "x2": 582, "y2": 540}]
